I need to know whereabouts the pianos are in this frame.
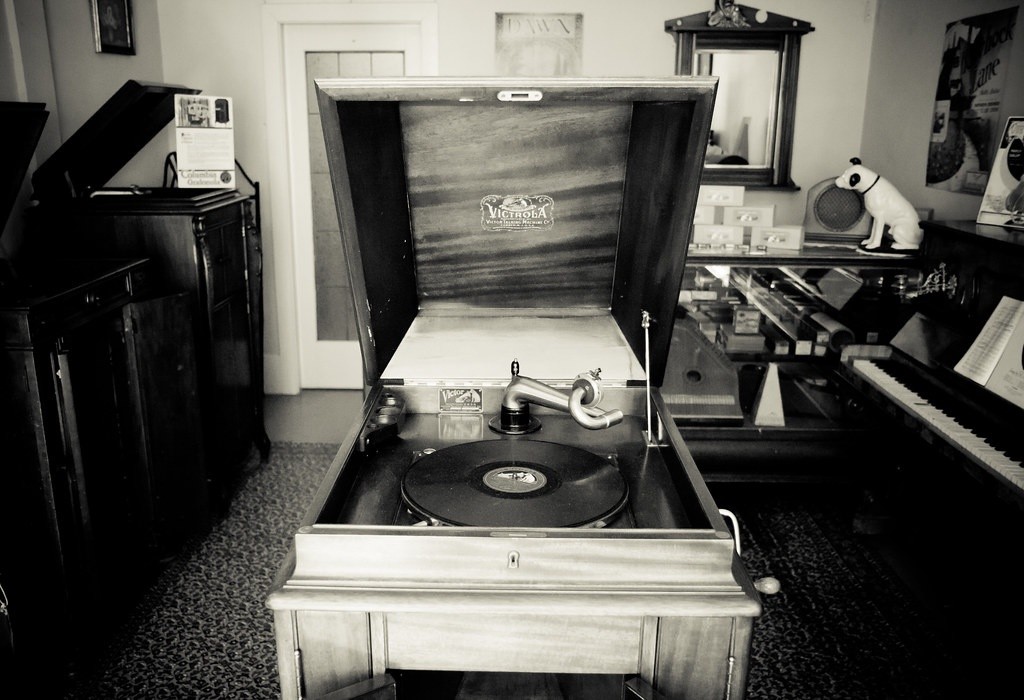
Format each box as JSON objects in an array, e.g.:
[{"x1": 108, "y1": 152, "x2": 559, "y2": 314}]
[{"x1": 820, "y1": 217, "x2": 1023, "y2": 615}]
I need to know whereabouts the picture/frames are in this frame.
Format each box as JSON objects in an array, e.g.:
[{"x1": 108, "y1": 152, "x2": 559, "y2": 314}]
[{"x1": 89, "y1": 1, "x2": 137, "y2": 54}]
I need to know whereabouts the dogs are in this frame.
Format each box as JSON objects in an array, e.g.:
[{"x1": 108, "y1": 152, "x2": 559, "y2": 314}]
[{"x1": 835, "y1": 157, "x2": 924, "y2": 250}]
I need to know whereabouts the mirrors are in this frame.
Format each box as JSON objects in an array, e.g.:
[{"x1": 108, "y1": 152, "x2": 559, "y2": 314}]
[{"x1": 664, "y1": 6, "x2": 811, "y2": 195}]
